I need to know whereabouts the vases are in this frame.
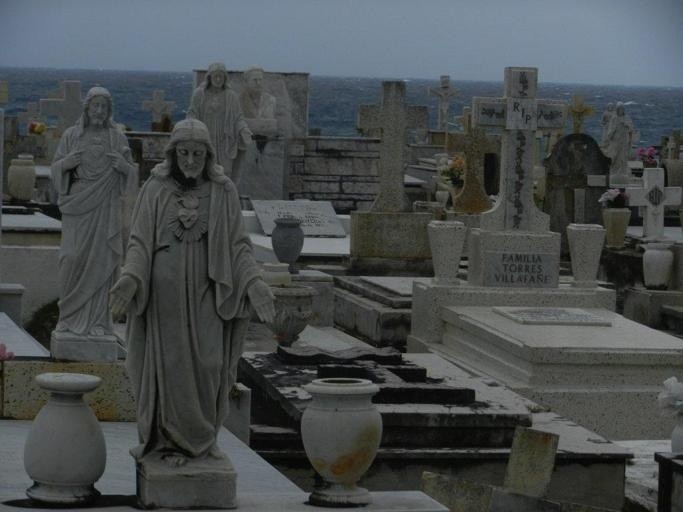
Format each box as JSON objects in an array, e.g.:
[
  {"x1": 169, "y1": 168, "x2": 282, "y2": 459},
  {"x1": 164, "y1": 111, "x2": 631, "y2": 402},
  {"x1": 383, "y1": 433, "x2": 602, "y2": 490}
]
[
  {"x1": 301, "y1": 377, "x2": 385, "y2": 506},
  {"x1": 643, "y1": 242, "x2": 675, "y2": 290},
  {"x1": 271, "y1": 217, "x2": 305, "y2": 273},
  {"x1": 601, "y1": 207, "x2": 632, "y2": 249},
  {"x1": 269, "y1": 282, "x2": 318, "y2": 344}
]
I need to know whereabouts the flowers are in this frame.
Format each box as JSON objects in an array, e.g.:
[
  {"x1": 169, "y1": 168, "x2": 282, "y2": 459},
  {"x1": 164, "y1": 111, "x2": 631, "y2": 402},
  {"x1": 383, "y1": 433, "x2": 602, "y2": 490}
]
[
  {"x1": 637, "y1": 147, "x2": 658, "y2": 167},
  {"x1": 597, "y1": 188, "x2": 629, "y2": 209},
  {"x1": 440, "y1": 158, "x2": 465, "y2": 188},
  {"x1": 28, "y1": 120, "x2": 46, "y2": 134}
]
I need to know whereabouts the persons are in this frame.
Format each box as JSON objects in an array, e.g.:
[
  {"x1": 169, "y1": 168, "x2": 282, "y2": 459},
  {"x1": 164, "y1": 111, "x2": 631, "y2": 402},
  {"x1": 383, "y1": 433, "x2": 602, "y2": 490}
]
[
  {"x1": 600, "y1": 103, "x2": 616, "y2": 143},
  {"x1": 431, "y1": 86, "x2": 460, "y2": 126},
  {"x1": 109, "y1": 118, "x2": 277, "y2": 467},
  {"x1": 607, "y1": 101, "x2": 633, "y2": 175},
  {"x1": 185, "y1": 61, "x2": 253, "y2": 179},
  {"x1": 237, "y1": 68, "x2": 277, "y2": 143},
  {"x1": 50, "y1": 86, "x2": 138, "y2": 336}
]
